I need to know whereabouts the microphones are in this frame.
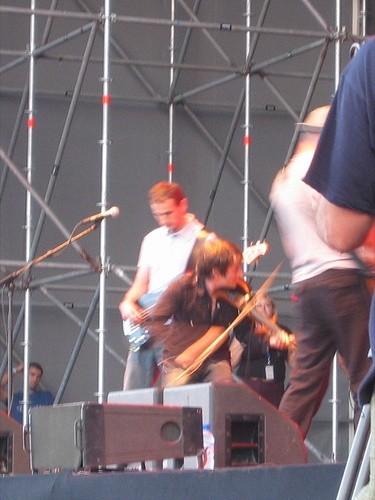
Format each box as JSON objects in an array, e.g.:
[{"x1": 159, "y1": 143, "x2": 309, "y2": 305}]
[{"x1": 81, "y1": 206, "x2": 119, "y2": 224}]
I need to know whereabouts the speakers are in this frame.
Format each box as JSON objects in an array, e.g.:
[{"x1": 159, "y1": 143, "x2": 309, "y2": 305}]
[{"x1": 21, "y1": 382, "x2": 307, "y2": 473}]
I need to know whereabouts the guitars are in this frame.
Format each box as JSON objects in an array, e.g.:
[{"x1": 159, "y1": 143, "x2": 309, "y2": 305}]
[{"x1": 123, "y1": 239, "x2": 270, "y2": 349}]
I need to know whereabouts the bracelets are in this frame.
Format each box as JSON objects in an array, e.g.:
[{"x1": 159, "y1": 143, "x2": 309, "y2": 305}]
[{"x1": 12, "y1": 368, "x2": 17, "y2": 374}]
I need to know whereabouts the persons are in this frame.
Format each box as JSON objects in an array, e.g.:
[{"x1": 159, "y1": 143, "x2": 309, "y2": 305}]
[
  {"x1": 144, "y1": 240, "x2": 297, "y2": 399},
  {"x1": 119, "y1": 181, "x2": 219, "y2": 391},
  {"x1": 271, "y1": 105, "x2": 373, "y2": 441},
  {"x1": 303, "y1": 32, "x2": 375, "y2": 411},
  {"x1": 0, "y1": 362, "x2": 55, "y2": 432}
]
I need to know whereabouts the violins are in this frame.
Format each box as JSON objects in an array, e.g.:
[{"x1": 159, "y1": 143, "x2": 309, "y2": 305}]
[{"x1": 212, "y1": 280, "x2": 298, "y2": 347}]
